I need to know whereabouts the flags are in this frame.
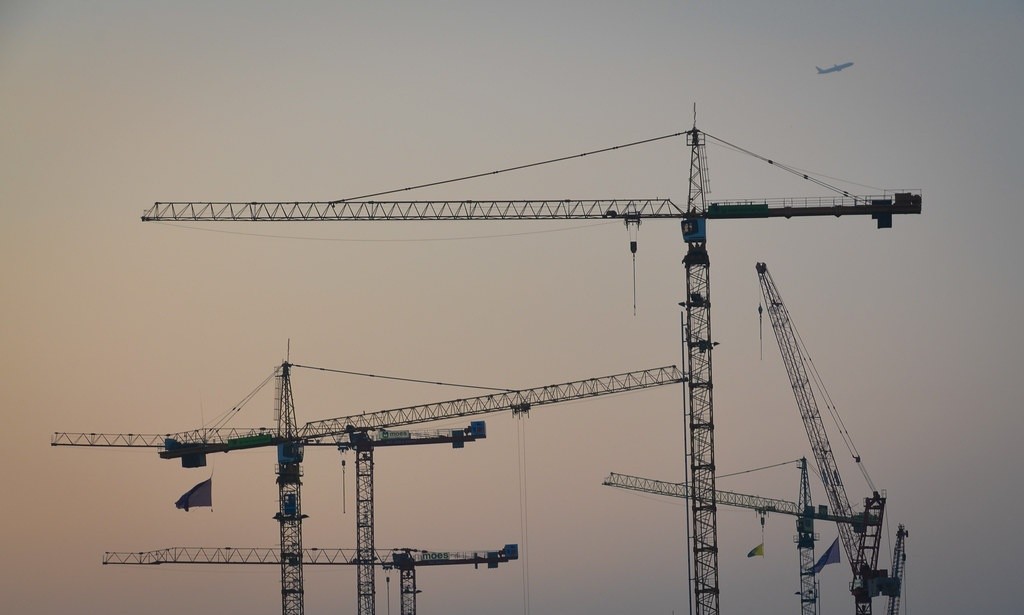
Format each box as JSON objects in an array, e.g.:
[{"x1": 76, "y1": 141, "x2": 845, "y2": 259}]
[
  {"x1": 809, "y1": 537, "x2": 840, "y2": 574},
  {"x1": 747, "y1": 543, "x2": 764, "y2": 558},
  {"x1": 175, "y1": 479, "x2": 212, "y2": 510}
]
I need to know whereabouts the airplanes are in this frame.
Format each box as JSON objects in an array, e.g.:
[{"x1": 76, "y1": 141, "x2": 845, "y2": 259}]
[{"x1": 815, "y1": 61, "x2": 854, "y2": 75}]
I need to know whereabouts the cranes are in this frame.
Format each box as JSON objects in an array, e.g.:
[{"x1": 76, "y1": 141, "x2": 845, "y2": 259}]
[
  {"x1": 99, "y1": 544, "x2": 519, "y2": 615},
  {"x1": 141, "y1": 100, "x2": 924, "y2": 615},
  {"x1": 158, "y1": 340, "x2": 691, "y2": 615},
  {"x1": 49, "y1": 419, "x2": 488, "y2": 615},
  {"x1": 600, "y1": 260, "x2": 909, "y2": 615}
]
